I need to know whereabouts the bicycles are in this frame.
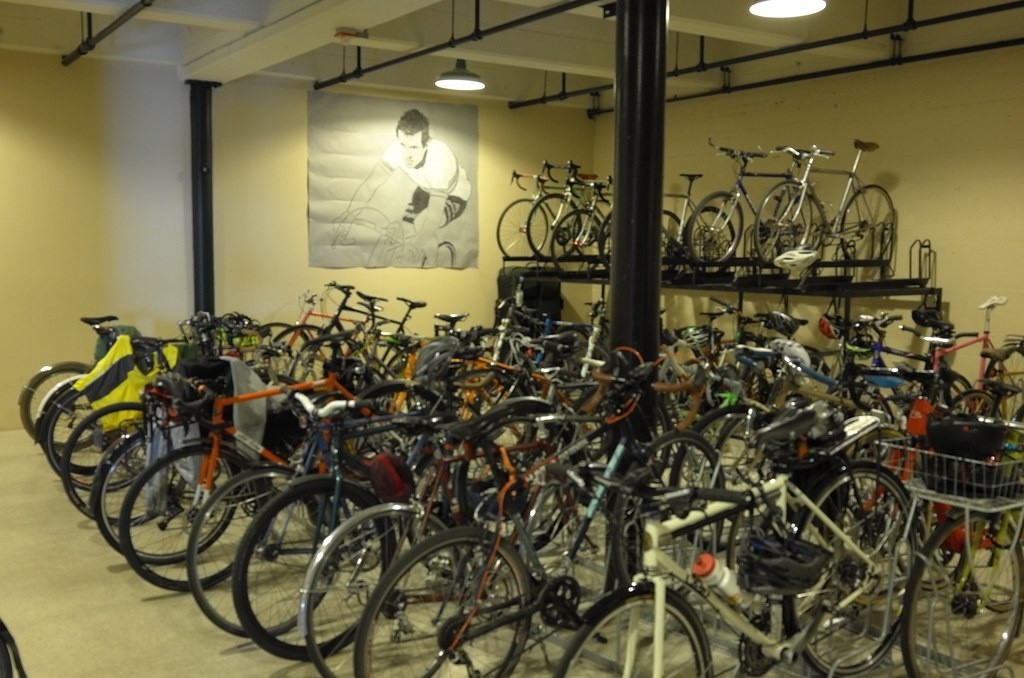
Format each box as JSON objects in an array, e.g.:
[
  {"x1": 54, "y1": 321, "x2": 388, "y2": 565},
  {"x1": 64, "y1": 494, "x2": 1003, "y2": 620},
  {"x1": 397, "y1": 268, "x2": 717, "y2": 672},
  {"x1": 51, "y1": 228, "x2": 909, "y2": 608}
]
[{"x1": 14, "y1": 123, "x2": 1023, "y2": 678}]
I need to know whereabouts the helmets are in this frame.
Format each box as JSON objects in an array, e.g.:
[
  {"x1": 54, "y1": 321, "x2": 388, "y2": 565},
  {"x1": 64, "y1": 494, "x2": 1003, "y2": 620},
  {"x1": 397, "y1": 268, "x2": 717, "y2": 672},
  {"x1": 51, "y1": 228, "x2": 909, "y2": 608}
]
[
  {"x1": 912, "y1": 306, "x2": 944, "y2": 327},
  {"x1": 766, "y1": 338, "x2": 811, "y2": 385},
  {"x1": 819, "y1": 314, "x2": 845, "y2": 339},
  {"x1": 774, "y1": 250, "x2": 819, "y2": 271},
  {"x1": 258, "y1": 407, "x2": 309, "y2": 460},
  {"x1": 734, "y1": 525, "x2": 833, "y2": 600},
  {"x1": 926, "y1": 410, "x2": 1006, "y2": 463},
  {"x1": 322, "y1": 356, "x2": 374, "y2": 394},
  {"x1": 413, "y1": 337, "x2": 461, "y2": 384},
  {"x1": 678, "y1": 325, "x2": 710, "y2": 350}
]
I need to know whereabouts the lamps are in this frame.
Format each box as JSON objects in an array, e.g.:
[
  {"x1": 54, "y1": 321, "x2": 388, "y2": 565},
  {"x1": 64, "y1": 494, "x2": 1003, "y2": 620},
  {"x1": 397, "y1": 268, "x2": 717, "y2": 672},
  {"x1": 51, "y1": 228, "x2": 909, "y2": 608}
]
[
  {"x1": 435, "y1": 59, "x2": 487, "y2": 92},
  {"x1": 748, "y1": 0, "x2": 827, "y2": 19}
]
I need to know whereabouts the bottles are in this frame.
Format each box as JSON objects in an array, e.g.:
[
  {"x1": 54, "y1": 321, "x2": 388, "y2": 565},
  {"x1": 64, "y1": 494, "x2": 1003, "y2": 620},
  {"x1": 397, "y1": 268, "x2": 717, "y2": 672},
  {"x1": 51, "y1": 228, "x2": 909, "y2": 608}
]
[
  {"x1": 1001, "y1": 442, "x2": 1023, "y2": 479},
  {"x1": 692, "y1": 554, "x2": 746, "y2": 609}
]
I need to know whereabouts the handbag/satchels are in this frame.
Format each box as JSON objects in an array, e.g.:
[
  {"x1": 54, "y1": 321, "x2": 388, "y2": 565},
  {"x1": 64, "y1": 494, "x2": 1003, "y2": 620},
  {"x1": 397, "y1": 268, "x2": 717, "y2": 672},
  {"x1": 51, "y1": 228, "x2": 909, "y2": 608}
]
[{"x1": 74, "y1": 334, "x2": 173, "y2": 431}]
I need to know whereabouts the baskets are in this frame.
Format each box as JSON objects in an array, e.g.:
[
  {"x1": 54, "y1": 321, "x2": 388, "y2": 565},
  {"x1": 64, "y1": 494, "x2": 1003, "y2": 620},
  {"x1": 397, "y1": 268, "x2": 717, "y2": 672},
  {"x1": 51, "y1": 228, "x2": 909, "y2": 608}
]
[{"x1": 875, "y1": 414, "x2": 1024, "y2": 506}]
